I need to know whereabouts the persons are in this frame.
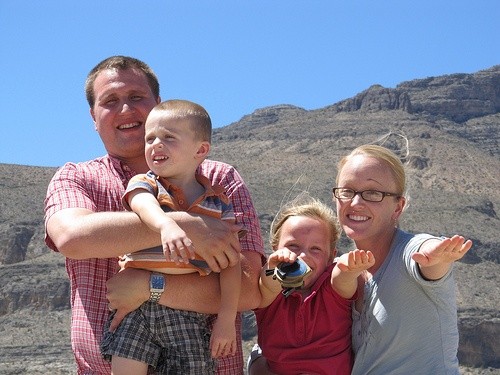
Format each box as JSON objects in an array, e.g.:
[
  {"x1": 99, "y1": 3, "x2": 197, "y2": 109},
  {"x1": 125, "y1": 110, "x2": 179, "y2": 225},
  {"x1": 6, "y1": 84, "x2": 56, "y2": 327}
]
[
  {"x1": 252, "y1": 200, "x2": 375, "y2": 375},
  {"x1": 247, "y1": 145, "x2": 472, "y2": 375},
  {"x1": 99, "y1": 100, "x2": 241, "y2": 375},
  {"x1": 43, "y1": 56, "x2": 266, "y2": 375}
]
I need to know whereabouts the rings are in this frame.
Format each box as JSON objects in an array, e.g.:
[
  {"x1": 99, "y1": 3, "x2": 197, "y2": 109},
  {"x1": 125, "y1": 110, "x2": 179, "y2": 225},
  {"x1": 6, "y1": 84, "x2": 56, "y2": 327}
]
[{"x1": 109, "y1": 305, "x2": 113, "y2": 312}]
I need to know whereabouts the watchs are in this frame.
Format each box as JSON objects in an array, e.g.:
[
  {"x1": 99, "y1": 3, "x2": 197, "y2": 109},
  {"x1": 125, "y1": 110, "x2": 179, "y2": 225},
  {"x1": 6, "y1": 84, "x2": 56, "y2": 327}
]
[{"x1": 147, "y1": 272, "x2": 166, "y2": 304}]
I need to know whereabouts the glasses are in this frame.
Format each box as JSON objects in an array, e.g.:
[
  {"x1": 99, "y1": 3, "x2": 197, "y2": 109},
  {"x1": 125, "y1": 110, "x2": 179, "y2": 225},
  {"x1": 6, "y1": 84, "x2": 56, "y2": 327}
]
[{"x1": 332, "y1": 187, "x2": 399, "y2": 203}]
